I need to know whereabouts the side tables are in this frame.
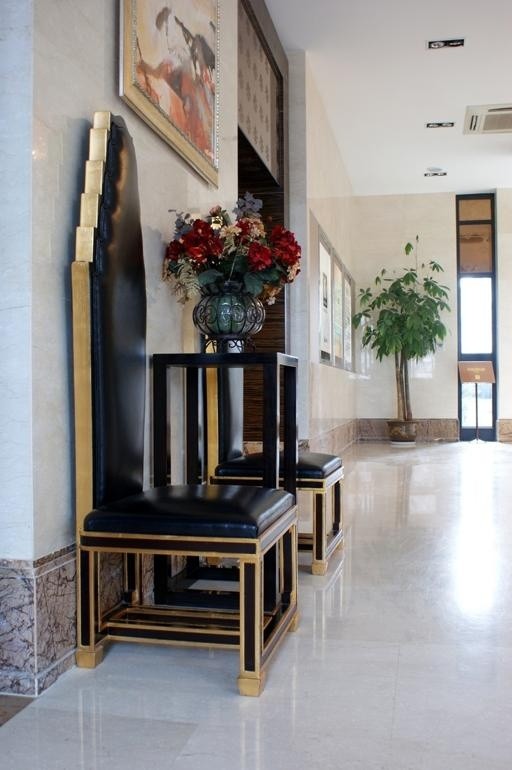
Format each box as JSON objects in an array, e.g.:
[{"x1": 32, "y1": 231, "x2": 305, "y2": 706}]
[{"x1": 149, "y1": 351, "x2": 302, "y2": 608}]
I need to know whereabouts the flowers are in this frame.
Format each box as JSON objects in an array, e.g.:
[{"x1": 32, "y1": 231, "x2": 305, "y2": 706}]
[{"x1": 162, "y1": 194, "x2": 304, "y2": 307}]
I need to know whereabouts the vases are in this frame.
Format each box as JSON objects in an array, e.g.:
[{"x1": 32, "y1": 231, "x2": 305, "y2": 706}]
[{"x1": 192, "y1": 278, "x2": 266, "y2": 353}]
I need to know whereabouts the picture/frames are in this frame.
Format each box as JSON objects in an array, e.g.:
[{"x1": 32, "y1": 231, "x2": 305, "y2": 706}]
[{"x1": 117, "y1": 2, "x2": 225, "y2": 190}]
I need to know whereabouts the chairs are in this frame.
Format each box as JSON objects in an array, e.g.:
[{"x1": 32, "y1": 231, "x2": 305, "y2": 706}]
[
  {"x1": 199, "y1": 334, "x2": 347, "y2": 575},
  {"x1": 70, "y1": 110, "x2": 299, "y2": 701}
]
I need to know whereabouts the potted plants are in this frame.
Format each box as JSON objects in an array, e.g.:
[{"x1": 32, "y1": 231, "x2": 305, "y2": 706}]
[{"x1": 351, "y1": 233, "x2": 453, "y2": 447}]
